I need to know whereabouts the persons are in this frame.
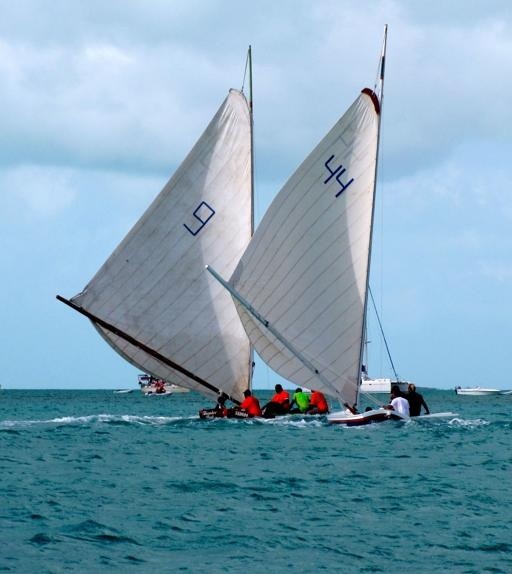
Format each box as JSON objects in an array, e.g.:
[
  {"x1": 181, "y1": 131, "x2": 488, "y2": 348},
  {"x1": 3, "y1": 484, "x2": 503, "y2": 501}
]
[
  {"x1": 288, "y1": 387, "x2": 310, "y2": 414},
  {"x1": 138, "y1": 373, "x2": 174, "y2": 394},
  {"x1": 405, "y1": 383, "x2": 431, "y2": 416},
  {"x1": 261, "y1": 383, "x2": 290, "y2": 415},
  {"x1": 233, "y1": 388, "x2": 263, "y2": 416},
  {"x1": 308, "y1": 389, "x2": 329, "y2": 414},
  {"x1": 382, "y1": 387, "x2": 410, "y2": 419},
  {"x1": 388, "y1": 384, "x2": 413, "y2": 416}
]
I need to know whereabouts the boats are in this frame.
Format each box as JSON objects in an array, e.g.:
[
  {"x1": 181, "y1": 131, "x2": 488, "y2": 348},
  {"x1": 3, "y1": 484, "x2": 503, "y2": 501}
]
[
  {"x1": 139, "y1": 374, "x2": 189, "y2": 394},
  {"x1": 456, "y1": 386, "x2": 500, "y2": 396},
  {"x1": 300, "y1": 363, "x2": 410, "y2": 394}
]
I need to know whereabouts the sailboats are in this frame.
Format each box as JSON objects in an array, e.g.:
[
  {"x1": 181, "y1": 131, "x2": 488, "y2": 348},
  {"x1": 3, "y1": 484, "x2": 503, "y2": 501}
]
[
  {"x1": 202, "y1": 24, "x2": 408, "y2": 424},
  {"x1": 56, "y1": 44, "x2": 254, "y2": 420}
]
